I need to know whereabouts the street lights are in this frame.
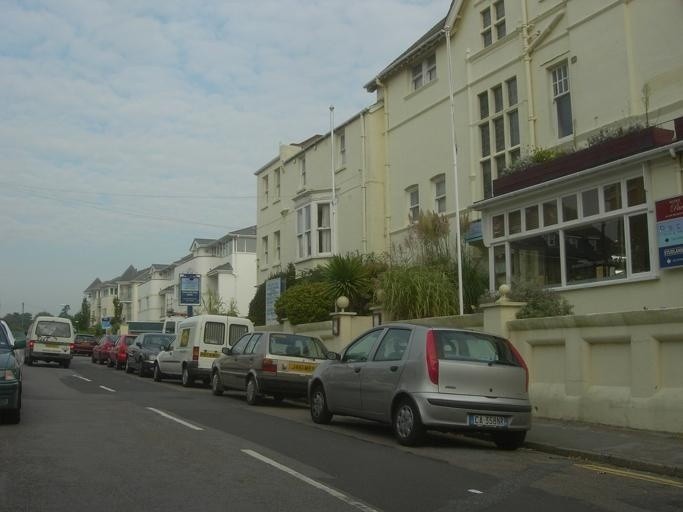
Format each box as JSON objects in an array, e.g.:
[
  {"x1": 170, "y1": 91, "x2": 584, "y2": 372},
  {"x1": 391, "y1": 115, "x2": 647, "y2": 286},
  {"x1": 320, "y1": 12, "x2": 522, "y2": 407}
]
[{"x1": 329, "y1": 104, "x2": 336, "y2": 256}]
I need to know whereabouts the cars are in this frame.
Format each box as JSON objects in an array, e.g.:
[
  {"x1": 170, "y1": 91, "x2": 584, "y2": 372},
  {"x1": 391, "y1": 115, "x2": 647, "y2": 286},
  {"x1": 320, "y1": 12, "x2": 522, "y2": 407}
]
[
  {"x1": 92, "y1": 335, "x2": 121, "y2": 364},
  {"x1": 74, "y1": 333, "x2": 98, "y2": 357},
  {"x1": 126, "y1": 332, "x2": 176, "y2": 375},
  {"x1": 106, "y1": 335, "x2": 139, "y2": 369},
  {"x1": 211, "y1": 331, "x2": 329, "y2": 406},
  {"x1": 0, "y1": 319, "x2": 27, "y2": 425},
  {"x1": 306, "y1": 323, "x2": 533, "y2": 449}
]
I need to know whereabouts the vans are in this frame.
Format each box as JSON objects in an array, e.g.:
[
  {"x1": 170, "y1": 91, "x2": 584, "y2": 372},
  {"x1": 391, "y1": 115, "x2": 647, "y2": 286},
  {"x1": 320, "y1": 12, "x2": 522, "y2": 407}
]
[{"x1": 24, "y1": 316, "x2": 75, "y2": 368}]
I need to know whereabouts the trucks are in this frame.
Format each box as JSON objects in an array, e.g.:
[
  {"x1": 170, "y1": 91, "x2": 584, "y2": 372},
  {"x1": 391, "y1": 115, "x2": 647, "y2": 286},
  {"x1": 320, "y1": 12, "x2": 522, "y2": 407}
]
[{"x1": 153, "y1": 314, "x2": 253, "y2": 388}]
